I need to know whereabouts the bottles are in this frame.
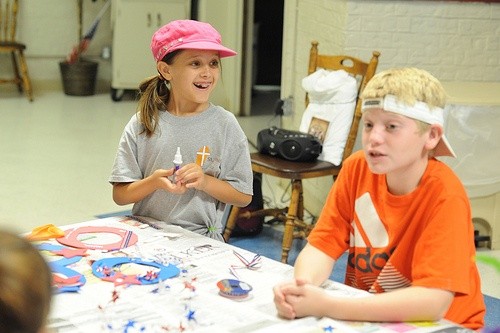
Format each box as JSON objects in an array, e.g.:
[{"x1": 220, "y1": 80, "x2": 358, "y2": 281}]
[{"x1": 172, "y1": 146, "x2": 183, "y2": 184}]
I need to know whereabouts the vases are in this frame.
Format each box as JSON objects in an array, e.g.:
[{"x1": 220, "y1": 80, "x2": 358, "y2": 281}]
[{"x1": 59, "y1": 58, "x2": 98, "y2": 96}]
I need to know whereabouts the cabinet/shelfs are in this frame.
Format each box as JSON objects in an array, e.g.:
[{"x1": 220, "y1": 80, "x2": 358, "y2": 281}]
[{"x1": 109, "y1": 0, "x2": 191, "y2": 101}]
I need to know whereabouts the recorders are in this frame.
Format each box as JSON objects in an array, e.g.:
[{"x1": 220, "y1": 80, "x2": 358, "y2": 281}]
[{"x1": 256, "y1": 126, "x2": 323, "y2": 161}]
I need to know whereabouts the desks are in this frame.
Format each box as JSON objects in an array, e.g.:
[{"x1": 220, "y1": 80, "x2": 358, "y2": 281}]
[{"x1": 19, "y1": 216, "x2": 477, "y2": 333}]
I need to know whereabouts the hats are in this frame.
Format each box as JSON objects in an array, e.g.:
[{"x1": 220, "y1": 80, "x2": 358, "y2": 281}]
[
  {"x1": 361, "y1": 94, "x2": 456, "y2": 157},
  {"x1": 151, "y1": 19, "x2": 237, "y2": 62}
]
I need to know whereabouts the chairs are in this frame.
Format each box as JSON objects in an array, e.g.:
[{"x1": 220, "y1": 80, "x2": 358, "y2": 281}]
[
  {"x1": 218, "y1": 39, "x2": 381, "y2": 263},
  {"x1": 0, "y1": 0, "x2": 34, "y2": 102}
]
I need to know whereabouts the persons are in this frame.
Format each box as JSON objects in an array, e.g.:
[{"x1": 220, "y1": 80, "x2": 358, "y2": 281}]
[
  {"x1": 274, "y1": 69, "x2": 487, "y2": 332},
  {"x1": 109, "y1": 19, "x2": 253, "y2": 244}
]
